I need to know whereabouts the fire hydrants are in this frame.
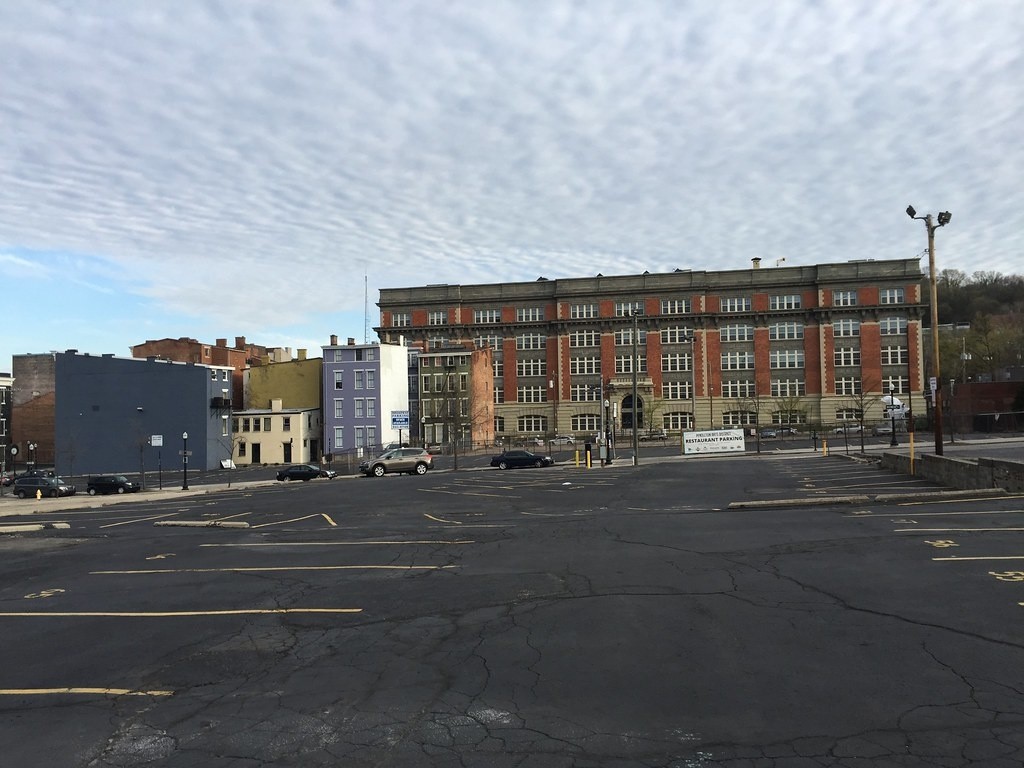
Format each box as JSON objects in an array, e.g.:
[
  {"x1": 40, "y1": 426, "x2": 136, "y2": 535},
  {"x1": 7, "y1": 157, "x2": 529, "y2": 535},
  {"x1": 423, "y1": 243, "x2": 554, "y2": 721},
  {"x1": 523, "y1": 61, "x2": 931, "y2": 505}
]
[{"x1": 36, "y1": 489, "x2": 42, "y2": 500}]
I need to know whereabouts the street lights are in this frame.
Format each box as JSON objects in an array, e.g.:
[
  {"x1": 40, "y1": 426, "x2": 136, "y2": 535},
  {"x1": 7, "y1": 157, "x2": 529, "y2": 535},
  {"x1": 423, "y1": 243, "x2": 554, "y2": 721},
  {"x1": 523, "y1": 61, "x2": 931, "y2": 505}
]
[
  {"x1": 889, "y1": 383, "x2": 899, "y2": 447},
  {"x1": 604, "y1": 399, "x2": 613, "y2": 465},
  {"x1": 182, "y1": 432, "x2": 190, "y2": 490},
  {"x1": 26, "y1": 440, "x2": 38, "y2": 477},
  {"x1": 906, "y1": 204, "x2": 952, "y2": 457}
]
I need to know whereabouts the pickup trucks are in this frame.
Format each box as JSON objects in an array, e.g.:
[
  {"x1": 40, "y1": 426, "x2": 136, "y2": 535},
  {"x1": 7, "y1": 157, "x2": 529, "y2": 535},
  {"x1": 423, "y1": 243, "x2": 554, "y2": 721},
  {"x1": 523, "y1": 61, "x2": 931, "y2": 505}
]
[{"x1": 547, "y1": 436, "x2": 585, "y2": 446}]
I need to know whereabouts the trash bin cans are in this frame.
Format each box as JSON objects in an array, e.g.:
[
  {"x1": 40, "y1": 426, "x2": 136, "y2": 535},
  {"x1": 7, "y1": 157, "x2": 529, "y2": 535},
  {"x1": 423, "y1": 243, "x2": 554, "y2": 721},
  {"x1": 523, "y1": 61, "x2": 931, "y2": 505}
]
[{"x1": 599, "y1": 444, "x2": 607, "y2": 460}]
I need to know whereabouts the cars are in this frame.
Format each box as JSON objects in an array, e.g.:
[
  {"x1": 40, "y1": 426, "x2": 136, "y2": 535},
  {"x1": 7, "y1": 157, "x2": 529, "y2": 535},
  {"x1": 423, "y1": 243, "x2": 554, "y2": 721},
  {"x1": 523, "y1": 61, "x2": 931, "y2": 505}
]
[
  {"x1": 494, "y1": 440, "x2": 504, "y2": 447},
  {"x1": 87, "y1": 475, "x2": 140, "y2": 495},
  {"x1": 871, "y1": 424, "x2": 893, "y2": 437},
  {"x1": 276, "y1": 464, "x2": 338, "y2": 482},
  {"x1": 638, "y1": 432, "x2": 668, "y2": 442},
  {"x1": 833, "y1": 423, "x2": 865, "y2": 434},
  {"x1": 490, "y1": 449, "x2": 555, "y2": 470},
  {"x1": 761, "y1": 430, "x2": 776, "y2": 438},
  {"x1": 776, "y1": 427, "x2": 798, "y2": 436},
  {"x1": 13, "y1": 477, "x2": 76, "y2": 497},
  {"x1": 514, "y1": 437, "x2": 539, "y2": 448},
  {"x1": 359, "y1": 447, "x2": 435, "y2": 477}
]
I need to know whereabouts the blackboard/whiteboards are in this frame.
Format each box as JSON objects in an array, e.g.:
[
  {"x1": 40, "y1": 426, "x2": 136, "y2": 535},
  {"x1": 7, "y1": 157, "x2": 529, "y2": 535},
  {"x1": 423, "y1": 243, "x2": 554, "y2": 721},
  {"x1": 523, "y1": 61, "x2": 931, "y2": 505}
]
[{"x1": 750, "y1": 429, "x2": 757, "y2": 436}]
